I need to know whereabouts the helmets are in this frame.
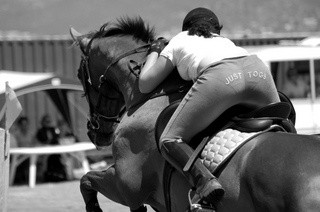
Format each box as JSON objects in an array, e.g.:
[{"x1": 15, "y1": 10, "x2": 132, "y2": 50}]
[{"x1": 182, "y1": 7, "x2": 221, "y2": 36}]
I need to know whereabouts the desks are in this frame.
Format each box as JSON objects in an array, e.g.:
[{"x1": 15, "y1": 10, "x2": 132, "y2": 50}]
[{"x1": 8, "y1": 142, "x2": 96, "y2": 188}]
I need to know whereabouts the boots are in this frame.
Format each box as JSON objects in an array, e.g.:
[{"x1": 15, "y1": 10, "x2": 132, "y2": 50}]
[{"x1": 160, "y1": 141, "x2": 225, "y2": 212}]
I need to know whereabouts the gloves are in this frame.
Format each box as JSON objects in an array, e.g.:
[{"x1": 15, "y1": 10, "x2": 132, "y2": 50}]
[{"x1": 147, "y1": 37, "x2": 168, "y2": 54}]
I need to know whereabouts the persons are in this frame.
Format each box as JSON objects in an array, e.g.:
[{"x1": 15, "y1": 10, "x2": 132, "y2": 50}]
[
  {"x1": 139, "y1": 7, "x2": 289, "y2": 212},
  {"x1": 10, "y1": 117, "x2": 41, "y2": 186},
  {"x1": 36, "y1": 114, "x2": 66, "y2": 183},
  {"x1": 283, "y1": 68, "x2": 309, "y2": 98}
]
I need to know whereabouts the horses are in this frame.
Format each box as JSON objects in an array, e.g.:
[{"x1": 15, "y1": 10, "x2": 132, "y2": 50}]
[{"x1": 70, "y1": 13, "x2": 320, "y2": 212}]
